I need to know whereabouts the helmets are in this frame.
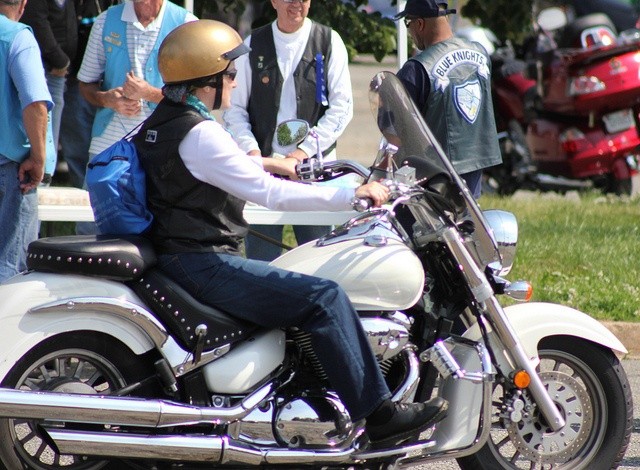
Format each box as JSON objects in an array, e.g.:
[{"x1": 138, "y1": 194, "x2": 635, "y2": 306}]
[{"x1": 157, "y1": 19, "x2": 251, "y2": 86}]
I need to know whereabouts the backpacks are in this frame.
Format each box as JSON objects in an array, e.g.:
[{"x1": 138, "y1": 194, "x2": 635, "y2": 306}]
[{"x1": 85, "y1": 139, "x2": 155, "y2": 236}]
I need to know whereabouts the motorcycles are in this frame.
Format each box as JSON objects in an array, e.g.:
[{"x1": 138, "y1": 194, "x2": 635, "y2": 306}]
[
  {"x1": 0, "y1": 71, "x2": 634, "y2": 470},
  {"x1": 488, "y1": 7, "x2": 640, "y2": 197}
]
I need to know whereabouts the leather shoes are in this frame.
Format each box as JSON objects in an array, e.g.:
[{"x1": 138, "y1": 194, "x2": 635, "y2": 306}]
[{"x1": 364, "y1": 396, "x2": 450, "y2": 449}]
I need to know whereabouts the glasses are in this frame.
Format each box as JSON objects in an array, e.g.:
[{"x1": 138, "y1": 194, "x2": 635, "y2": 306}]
[
  {"x1": 403, "y1": 16, "x2": 418, "y2": 28},
  {"x1": 223, "y1": 68, "x2": 237, "y2": 82}
]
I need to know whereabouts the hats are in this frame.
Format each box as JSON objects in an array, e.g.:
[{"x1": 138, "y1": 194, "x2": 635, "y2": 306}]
[{"x1": 391, "y1": 0, "x2": 456, "y2": 21}]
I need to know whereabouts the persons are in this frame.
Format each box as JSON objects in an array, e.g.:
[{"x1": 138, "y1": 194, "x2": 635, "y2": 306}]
[
  {"x1": 131, "y1": 18, "x2": 450, "y2": 450},
  {"x1": 223, "y1": 0, "x2": 354, "y2": 261},
  {"x1": 377, "y1": 1, "x2": 503, "y2": 197},
  {"x1": 73, "y1": 0, "x2": 201, "y2": 234},
  {"x1": 23, "y1": 0, "x2": 78, "y2": 187},
  {"x1": 61, "y1": 0, "x2": 117, "y2": 188},
  {"x1": 0, "y1": 1, "x2": 57, "y2": 281}
]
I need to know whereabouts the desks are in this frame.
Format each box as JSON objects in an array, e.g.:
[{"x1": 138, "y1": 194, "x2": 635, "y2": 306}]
[{"x1": 37, "y1": 185, "x2": 359, "y2": 227}]
